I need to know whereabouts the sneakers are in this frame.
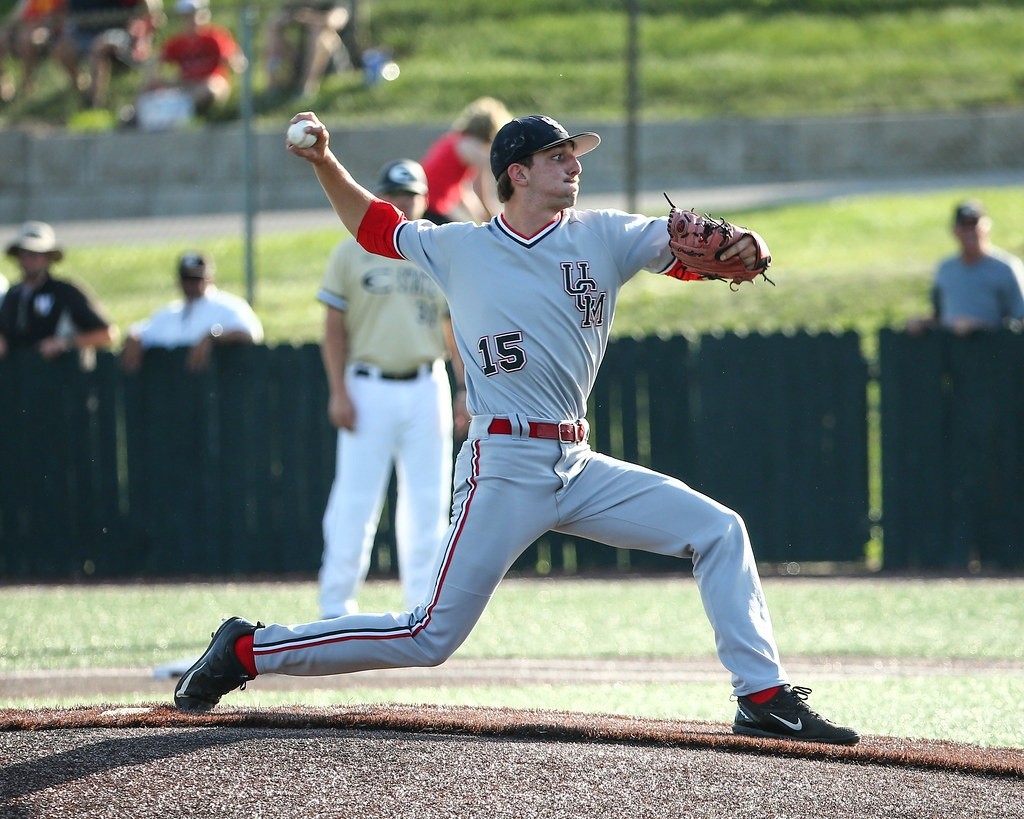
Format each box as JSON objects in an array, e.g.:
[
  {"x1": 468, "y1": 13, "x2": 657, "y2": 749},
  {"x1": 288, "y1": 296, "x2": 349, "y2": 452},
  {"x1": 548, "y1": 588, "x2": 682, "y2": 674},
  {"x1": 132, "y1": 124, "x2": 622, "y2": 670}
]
[
  {"x1": 173, "y1": 615, "x2": 264, "y2": 712},
  {"x1": 731, "y1": 684, "x2": 862, "y2": 746}
]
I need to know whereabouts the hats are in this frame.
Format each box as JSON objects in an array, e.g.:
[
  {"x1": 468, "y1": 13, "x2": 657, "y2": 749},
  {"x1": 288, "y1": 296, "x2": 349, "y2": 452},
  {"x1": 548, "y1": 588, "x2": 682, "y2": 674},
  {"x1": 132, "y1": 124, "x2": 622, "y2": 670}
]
[
  {"x1": 374, "y1": 158, "x2": 429, "y2": 197},
  {"x1": 178, "y1": 254, "x2": 208, "y2": 283},
  {"x1": 956, "y1": 206, "x2": 983, "y2": 223},
  {"x1": 6, "y1": 221, "x2": 62, "y2": 261},
  {"x1": 489, "y1": 116, "x2": 602, "y2": 181}
]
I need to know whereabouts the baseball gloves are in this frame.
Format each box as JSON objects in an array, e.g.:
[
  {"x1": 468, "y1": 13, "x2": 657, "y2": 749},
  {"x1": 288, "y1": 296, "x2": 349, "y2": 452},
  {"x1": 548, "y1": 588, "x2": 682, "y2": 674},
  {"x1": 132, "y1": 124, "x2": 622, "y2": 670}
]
[{"x1": 667, "y1": 207, "x2": 773, "y2": 285}]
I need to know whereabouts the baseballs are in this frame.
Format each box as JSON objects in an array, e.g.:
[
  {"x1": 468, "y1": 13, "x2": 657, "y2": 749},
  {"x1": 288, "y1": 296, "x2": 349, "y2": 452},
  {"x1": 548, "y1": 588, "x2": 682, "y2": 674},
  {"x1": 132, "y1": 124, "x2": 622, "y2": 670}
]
[{"x1": 287, "y1": 119, "x2": 318, "y2": 149}]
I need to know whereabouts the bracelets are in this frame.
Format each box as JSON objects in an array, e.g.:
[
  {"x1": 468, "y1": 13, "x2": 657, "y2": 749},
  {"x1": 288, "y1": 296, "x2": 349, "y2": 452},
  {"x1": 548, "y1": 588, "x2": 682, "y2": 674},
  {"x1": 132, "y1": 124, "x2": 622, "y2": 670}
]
[
  {"x1": 455, "y1": 384, "x2": 466, "y2": 392},
  {"x1": 65, "y1": 333, "x2": 74, "y2": 352}
]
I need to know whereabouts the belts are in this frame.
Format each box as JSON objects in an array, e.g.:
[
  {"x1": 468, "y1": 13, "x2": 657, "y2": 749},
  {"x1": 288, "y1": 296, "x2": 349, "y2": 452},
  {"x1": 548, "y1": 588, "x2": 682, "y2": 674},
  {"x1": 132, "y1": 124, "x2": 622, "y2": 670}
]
[
  {"x1": 487, "y1": 417, "x2": 584, "y2": 444},
  {"x1": 355, "y1": 369, "x2": 418, "y2": 381}
]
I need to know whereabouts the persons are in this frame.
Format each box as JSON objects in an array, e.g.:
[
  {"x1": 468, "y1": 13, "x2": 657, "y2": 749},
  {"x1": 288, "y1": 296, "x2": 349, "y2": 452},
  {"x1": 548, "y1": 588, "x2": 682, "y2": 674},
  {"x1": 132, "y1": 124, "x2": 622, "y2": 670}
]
[
  {"x1": 1, "y1": 220, "x2": 117, "y2": 365},
  {"x1": 122, "y1": 249, "x2": 263, "y2": 369},
  {"x1": 319, "y1": 158, "x2": 472, "y2": 620},
  {"x1": 419, "y1": 95, "x2": 511, "y2": 223},
  {"x1": 909, "y1": 202, "x2": 1024, "y2": 331},
  {"x1": 0, "y1": 0, "x2": 377, "y2": 114},
  {"x1": 174, "y1": 112, "x2": 857, "y2": 745}
]
